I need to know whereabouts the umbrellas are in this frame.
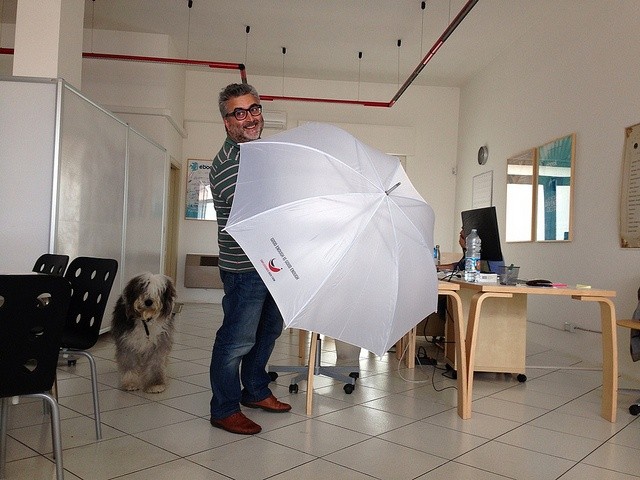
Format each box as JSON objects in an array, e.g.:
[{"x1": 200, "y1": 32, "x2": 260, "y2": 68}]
[{"x1": 221, "y1": 121, "x2": 438, "y2": 361}]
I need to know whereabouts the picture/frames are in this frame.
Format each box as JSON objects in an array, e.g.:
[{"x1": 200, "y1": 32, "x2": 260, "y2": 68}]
[
  {"x1": 505, "y1": 148, "x2": 535, "y2": 243},
  {"x1": 184, "y1": 158, "x2": 217, "y2": 221},
  {"x1": 535, "y1": 131, "x2": 575, "y2": 243}
]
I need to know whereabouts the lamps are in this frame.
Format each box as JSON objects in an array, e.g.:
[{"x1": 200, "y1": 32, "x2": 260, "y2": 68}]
[{"x1": 1, "y1": 2, "x2": 480, "y2": 107}]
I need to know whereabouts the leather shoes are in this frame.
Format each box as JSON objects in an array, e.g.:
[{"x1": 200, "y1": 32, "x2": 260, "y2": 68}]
[
  {"x1": 240, "y1": 393, "x2": 292, "y2": 412},
  {"x1": 210, "y1": 410, "x2": 261, "y2": 434}
]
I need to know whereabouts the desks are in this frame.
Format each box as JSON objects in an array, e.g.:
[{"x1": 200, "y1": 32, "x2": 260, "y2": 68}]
[
  {"x1": 298, "y1": 280, "x2": 462, "y2": 422},
  {"x1": 453, "y1": 274, "x2": 620, "y2": 423}
]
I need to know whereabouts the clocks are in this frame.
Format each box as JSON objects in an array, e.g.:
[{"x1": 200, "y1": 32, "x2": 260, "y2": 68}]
[{"x1": 477, "y1": 146, "x2": 487, "y2": 165}]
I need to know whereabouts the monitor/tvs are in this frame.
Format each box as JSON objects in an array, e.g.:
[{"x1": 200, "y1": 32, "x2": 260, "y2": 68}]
[{"x1": 460, "y1": 206, "x2": 503, "y2": 274}]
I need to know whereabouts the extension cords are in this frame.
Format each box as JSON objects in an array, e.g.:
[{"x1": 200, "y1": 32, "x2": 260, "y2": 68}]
[
  {"x1": 416, "y1": 355, "x2": 437, "y2": 365},
  {"x1": 434, "y1": 338, "x2": 446, "y2": 349}
]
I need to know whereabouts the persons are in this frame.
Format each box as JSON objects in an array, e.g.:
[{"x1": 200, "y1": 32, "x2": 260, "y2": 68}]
[{"x1": 210, "y1": 83, "x2": 292, "y2": 434}]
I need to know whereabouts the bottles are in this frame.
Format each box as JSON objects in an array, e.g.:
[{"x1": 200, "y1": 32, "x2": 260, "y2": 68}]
[{"x1": 465, "y1": 229, "x2": 482, "y2": 282}]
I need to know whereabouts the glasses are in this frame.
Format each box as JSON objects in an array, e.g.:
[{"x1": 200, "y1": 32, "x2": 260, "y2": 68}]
[{"x1": 226, "y1": 104, "x2": 263, "y2": 121}]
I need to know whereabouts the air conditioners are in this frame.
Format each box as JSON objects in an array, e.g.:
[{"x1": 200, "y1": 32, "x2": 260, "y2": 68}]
[{"x1": 261, "y1": 109, "x2": 286, "y2": 130}]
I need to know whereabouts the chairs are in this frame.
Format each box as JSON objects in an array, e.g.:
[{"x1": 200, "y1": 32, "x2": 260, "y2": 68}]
[
  {"x1": 0, "y1": 274, "x2": 71, "y2": 479},
  {"x1": 32, "y1": 255, "x2": 69, "y2": 279},
  {"x1": 615, "y1": 285, "x2": 640, "y2": 416},
  {"x1": 43, "y1": 256, "x2": 118, "y2": 442}
]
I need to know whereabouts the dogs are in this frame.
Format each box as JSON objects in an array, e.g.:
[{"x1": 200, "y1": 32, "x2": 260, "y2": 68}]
[{"x1": 111, "y1": 271, "x2": 185, "y2": 395}]
